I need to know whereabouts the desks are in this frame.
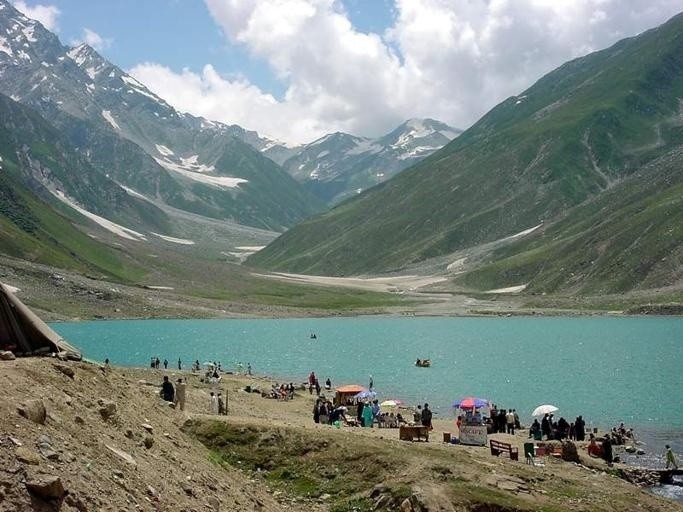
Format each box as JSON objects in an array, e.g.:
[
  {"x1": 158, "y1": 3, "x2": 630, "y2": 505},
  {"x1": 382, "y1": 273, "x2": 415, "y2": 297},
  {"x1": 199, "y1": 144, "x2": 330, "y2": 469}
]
[
  {"x1": 400, "y1": 424, "x2": 429, "y2": 442},
  {"x1": 459, "y1": 425, "x2": 487, "y2": 447}
]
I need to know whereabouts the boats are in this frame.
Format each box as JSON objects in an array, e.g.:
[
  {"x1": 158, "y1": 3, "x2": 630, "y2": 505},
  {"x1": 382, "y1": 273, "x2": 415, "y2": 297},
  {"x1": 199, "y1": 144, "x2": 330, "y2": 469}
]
[
  {"x1": 311, "y1": 333, "x2": 316, "y2": 338},
  {"x1": 415, "y1": 358, "x2": 431, "y2": 367}
]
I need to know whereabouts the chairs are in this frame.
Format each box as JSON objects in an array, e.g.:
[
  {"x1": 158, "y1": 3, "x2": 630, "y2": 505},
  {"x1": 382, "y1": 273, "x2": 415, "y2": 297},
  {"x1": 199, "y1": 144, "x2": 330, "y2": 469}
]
[
  {"x1": 549, "y1": 448, "x2": 563, "y2": 471},
  {"x1": 527, "y1": 452, "x2": 545, "y2": 474}
]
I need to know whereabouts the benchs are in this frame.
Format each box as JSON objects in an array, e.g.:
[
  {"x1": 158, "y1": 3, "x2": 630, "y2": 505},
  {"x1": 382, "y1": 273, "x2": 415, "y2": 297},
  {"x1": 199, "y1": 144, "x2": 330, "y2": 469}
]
[{"x1": 490, "y1": 440, "x2": 518, "y2": 461}]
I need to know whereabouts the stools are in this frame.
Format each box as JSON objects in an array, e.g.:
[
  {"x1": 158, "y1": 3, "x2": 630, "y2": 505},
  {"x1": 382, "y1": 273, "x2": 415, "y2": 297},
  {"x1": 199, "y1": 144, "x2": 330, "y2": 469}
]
[{"x1": 443, "y1": 432, "x2": 451, "y2": 443}]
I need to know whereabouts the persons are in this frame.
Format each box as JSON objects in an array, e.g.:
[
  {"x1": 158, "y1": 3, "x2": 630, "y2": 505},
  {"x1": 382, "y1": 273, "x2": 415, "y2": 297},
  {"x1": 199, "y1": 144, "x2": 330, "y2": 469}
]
[
  {"x1": 665, "y1": 444, "x2": 678, "y2": 469},
  {"x1": 313, "y1": 375, "x2": 433, "y2": 434},
  {"x1": 457, "y1": 403, "x2": 522, "y2": 435},
  {"x1": 151, "y1": 356, "x2": 253, "y2": 415},
  {"x1": 589, "y1": 422, "x2": 634, "y2": 465},
  {"x1": 528, "y1": 413, "x2": 586, "y2": 441},
  {"x1": 416, "y1": 358, "x2": 430, "y2": 364},
  {"x1": 253, "y1": 371, "x2": 331, "y2": 401}
]
